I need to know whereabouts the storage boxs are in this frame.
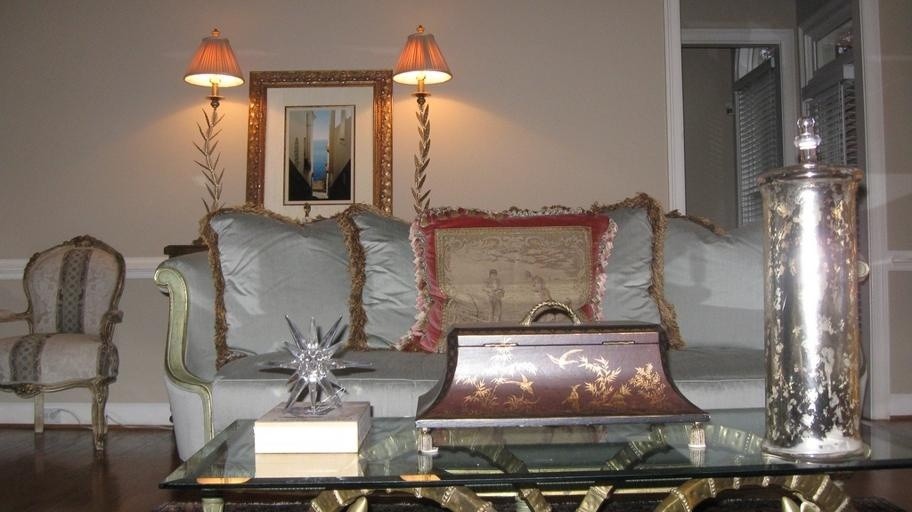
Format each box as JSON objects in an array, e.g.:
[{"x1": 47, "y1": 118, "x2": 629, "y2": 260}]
[{"x1": 415, "y1": 322, "x2": 709, "y2": 423}]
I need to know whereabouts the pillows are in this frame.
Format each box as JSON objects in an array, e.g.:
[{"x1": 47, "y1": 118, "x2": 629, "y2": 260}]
[
  {"x1": 400, "y1": 206, "x2": 619, "y2": 353},
  {"x1": 199, "y1": 205, "x2": 352, "y2": 372},
  {"x1": 592, "y1": 192, "x2": 685, "y2": 353},
  {"x1": 343, "y1": 203, "x2": 420, "y2": 351}
]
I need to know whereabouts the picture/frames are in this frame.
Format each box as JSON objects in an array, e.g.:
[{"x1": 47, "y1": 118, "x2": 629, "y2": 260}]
[{"x1": 248, "y1": 67, "x2": 391, "y2": 225}]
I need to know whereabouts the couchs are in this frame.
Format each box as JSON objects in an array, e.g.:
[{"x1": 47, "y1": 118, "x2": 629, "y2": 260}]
[{"x1": 147, "y1": 207, "x2": 890, "y2": 509}]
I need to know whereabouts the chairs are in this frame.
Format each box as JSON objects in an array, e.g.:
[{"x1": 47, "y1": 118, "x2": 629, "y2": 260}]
[{"x1": 2, "y1": 235, "x2": 127, "y2": 464}]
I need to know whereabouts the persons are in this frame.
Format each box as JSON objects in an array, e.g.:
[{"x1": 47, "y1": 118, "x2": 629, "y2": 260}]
[
  {"x1": 522, "y1": 269, "x2": 560, "y2": 321},
  {"x1": 481, "y1": 268, "x2": 504, "y2": 321}
]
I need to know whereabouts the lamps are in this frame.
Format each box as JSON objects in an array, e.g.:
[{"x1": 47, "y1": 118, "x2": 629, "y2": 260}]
[
  {"x1": 391, "y1": 26, "x2": 453, "y2": 218},
  {"x1": 183, "y1": 27, "x2": 245, "y2": 216}
]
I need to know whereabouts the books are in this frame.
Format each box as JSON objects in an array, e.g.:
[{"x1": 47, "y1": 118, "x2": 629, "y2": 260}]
[
  {"x1": 254, "y1": 437, "x2": 374, "y2": 480},
  {"x1": 253, "y1": 400, "x2": 375, "y2": 452}
]
